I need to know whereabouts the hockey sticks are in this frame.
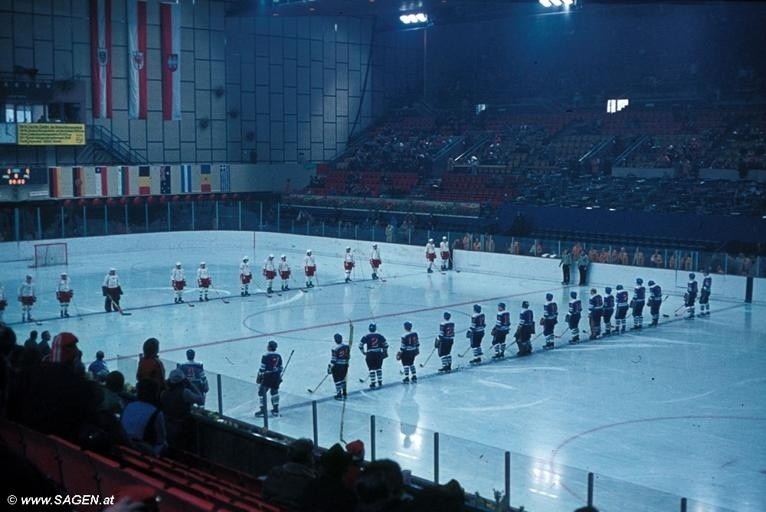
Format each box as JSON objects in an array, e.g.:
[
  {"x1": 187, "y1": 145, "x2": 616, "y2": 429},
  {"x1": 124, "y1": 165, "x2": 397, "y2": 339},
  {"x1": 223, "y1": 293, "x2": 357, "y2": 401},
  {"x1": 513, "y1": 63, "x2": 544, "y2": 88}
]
[
  {"x1": 400, "y1": 370, "x2": 405, "y2": 374},
  {"x1": 359, "y1": 375, "x2": 370, "y2": 383},
  {"x1": 72, "y1": 297, "x2": 83, "y2": 320},
  {"x1": 307, "y1": 372, "x2": 329, "y2": 393},
  {"x1": 583, "y1": 329, "x2": 590, "y2": 333},
  {"x1": 430, "y1": 261, "x2": 446, "y2": 274},
  {"x1": 316, "y1": 273, "x2": 322, "y2": 291},
  {"x1": 175, "y1": 286, "x2": 195, "y2": 307},
  {"x1": 290, "y1": 274, "x2": 308, "y2": 293},
  {"x1": 252, "y1": 279, "x2": 272, "y2": 297},
  {"x1": 107, "y1": 294, "x2": 132, "y2": 315},
  {"x1": 611, "y1": 314, "x2": 632, "y2": 328},
  {"x1": 255, "y1": 276, "x2": 282, "y2": 296},
  {"x1": 555, "y1": 327, "x2": 570, "y2": 338},
  {"x1": 663, "y1": 302, "x2": 684, "y2": 317},
  {"x1": 21, "y1": 301, "x2": 42, "y2": 326},
  {"x1": 375, "y1": 268, "x2": 386, "y2": 282},
  {"x1": 353, "y1": 266, "x2": 357, "y2": 286},
  {"x1": 211, "y1": 284, "x2": 229, "y2": 303},
  {"x1": 458, "y1": 346, "x2": 470, "y2": 357},
  {"x1": 420, "y1": 345, "x2": 436, "y2": 367},
  {"x1": 449, "y1": 257, "x2": 461, "y2": 273},
  {"x1": 675, "y1": 297, "x2": 700, "y2": 316}
]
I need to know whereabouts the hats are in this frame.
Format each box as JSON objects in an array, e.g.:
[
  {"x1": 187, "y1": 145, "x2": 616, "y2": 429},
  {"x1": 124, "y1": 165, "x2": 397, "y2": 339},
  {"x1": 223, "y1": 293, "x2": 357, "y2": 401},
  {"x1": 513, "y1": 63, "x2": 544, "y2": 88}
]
[{"x1": 169, "y1": 369, "x2": 186, "y2": 384}]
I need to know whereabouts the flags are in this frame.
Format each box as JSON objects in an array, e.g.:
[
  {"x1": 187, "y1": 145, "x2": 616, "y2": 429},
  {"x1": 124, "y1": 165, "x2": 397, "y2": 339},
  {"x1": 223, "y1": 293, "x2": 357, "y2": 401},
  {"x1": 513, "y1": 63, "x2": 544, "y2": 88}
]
[
  {"x1": 220, "y1": 164, "x2": 231, "y2": 193},
  {"x1": 201, "y1": 165, "x2": 212, "y2": 192},
  {"x1": 48, "y1": 167, "x2": 62, "y2": 197},
  {"x1": 118, "y1": 164, "x2": 129, "y2": 197},
  {"x1": 159, "y1": 165, "x2": 172, "y2": 193},
  {"x1": 94, "y1": 166, "x2": 108, "y2": 197},
  {"x1": 71, "y1": 167, "x2": 85, "y2": 197},
  {"x1": 181, "y1": 164, "x2": 192, "y2": 193},
  {"x1": 138, "y1": 166, "x2": 151, "y2": 194}
]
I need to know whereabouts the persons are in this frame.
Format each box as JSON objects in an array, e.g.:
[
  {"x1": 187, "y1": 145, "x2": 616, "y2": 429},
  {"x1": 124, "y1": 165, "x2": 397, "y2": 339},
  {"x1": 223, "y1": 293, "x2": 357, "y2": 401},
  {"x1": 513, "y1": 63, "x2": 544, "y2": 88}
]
[
  {"x1": 611, "y1": 285, "x2": 628, "y2": 334},
  {"x1": 629, "y1": 278, "x2": 646, "y2": 331},
  {"x1": 342, "y1": 246, "x2": 355, "y2": 283},
  {"x1": 195, "y1": 262, "x2": 212, "y2": 301},
  {"x1": 489, "y1": 303, "x2": 510, "y2": 362},
  {"x1": 277, "y1": 255, "x2": 291, "y2": 290},
  {"x1": 440, "y1": 237, "x2": 453, "y2": 272},
  {"x1": 368, "y1": 242, "x2": 382, "y2": 280},
  {"x1": 103, "y1": 266, "x2": 126, "y2": 311},
  {"x1": 425, "y1": 238, "x2": 437, "y2": 271},
  {"x1": 588, "y1": 287, "x2": 604, "y2": 339},
  {"x1": 682, "y1": 273, "x2": 698, "y2": 320},
  {"x1": 647, "y1": 281, "x2": 662, "y2": 325},
  {"x1": 466, "y1": 304, "x2": 486, "y2": 365},
  {"x1": 302, "y1": 132, "x2": 447, "y2": 201},
  {"x1": 456, "y1": 65, "x2": 766, "y2": 275},
  {"x1": 558, "y1": 247, "x2": 572, "y2": 286},
  {"x1": 359, "y1": 323, "x2": 389, "y2": 389},
  {"x1": 602, "y1": 287, "x2": 615, "y2": 336},
  {"x1": 566, "y1": 291, "x2": 582, "y2": 344},
  {"x1": 576, "y1": 247, "x2": 590, "y2": 285},
  {"x1": 18, "y1": 275, "x2": 38, "y2": 322},
  {"x1": 238, "y1": 257, "x2": 253, "y2": 295},
  {"x1": 540, "y1": 293, "x2": 558, "y2": 349},
  {"x1": 396, "y1": 321, "x2": 421, "y2": 384},
  {"x1": 697, "y1": 272, "x2": 712, "y2": 316},
  {"x1": 56, "y1": 273, "x2": 74, "y2": 317},
  {"x1": 253, "y1": 340, "x2": 283, "y2": 419},
  {"x1": 264, "y1": 432, "x2": 600, "y2": 512},
  {"x1": 290, "y1": 210, "x2": 417, "y2": 244},
  {"x1": 435, "y1": 311, "x2": 456, "y2": 372},
  {"x1": 303, "y1": 249, "x2": 317, "y2": 287},
  {"x1": 0, "y1": 285, "x2": 9, "y2": 314},
  {"x1": 513, "y1": 300, "x2": 534, "y2": 356},
  {"x1": 2, "y1": 339, "x2": 207, "y2": 512},
  {"x1": 170, "y1": 262, "x2": 187, "y2": 302},
  {"x1": 263, "y1": 255, "x2": 276, "y2": 293},
  {"x1": 326, "y1": 333, "x2": 351, "y2": 400}
]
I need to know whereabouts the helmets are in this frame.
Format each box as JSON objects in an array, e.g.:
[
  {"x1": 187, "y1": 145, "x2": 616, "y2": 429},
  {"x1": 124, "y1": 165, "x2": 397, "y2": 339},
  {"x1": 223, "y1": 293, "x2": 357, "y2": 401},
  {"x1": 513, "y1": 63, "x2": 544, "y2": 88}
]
[
  {"x1": 186, "y1": 350, "x2": 195, "y2": 360},
  {"x1": 267, "y1": 341, "x2": 278, "y2": 351},
  {"x1": 689, "y1": 269, "x2": 710, "y2": 280},
  {"x1": 26, "y1": 267, "x2": 116, "y2": 279},
  {"x1": 243, "y1": 249, "x2": 312, "y2": 262},
  {"x1": 429, "y1": 236, "x2": 447, "y2": 242},
  {"x1": 176, "y1": 262, "x2": 206, "y2": 266},
  {"x1": 346, "y1": 243, "x2": 377, "y2": 251}
]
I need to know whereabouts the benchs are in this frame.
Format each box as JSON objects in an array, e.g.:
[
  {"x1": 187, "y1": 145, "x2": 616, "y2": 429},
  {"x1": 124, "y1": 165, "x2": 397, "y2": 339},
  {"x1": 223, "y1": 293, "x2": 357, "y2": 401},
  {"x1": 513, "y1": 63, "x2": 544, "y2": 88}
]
[{"x1": 1, "y1": 421, "x2": 283, "y2": 511}]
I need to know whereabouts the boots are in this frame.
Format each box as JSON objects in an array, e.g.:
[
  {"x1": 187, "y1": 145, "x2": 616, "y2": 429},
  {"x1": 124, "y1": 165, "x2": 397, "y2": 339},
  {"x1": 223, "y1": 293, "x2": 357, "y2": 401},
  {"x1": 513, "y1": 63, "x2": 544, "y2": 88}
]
[
  {"x1": 174, "y1": 297, "x2": 209, "y2": 304},
  {"x1": 428, "y1": 265, "x2": 448, "y2": 273},
  {"x1": 686, "y1": 311, "x2": 710, "y2": 319},
  {"x1": 334, "y1": 351, "x2": 505, "y2": 398},
  {"x1": 241, "y1": 281, "x2": 314, "y2": 296},
  {"x1": 271, "y1": 404, "x2": 278, "y2": 412},
  {"x1": 22, "y1": 310, "x2": 69, "y2": 323},
  {"x1": 255, "y1": 406, "x2": 264, "y2": 415},
  {"x1": 345, "y1": 273, "x2": 378, "y2": 283}
]
